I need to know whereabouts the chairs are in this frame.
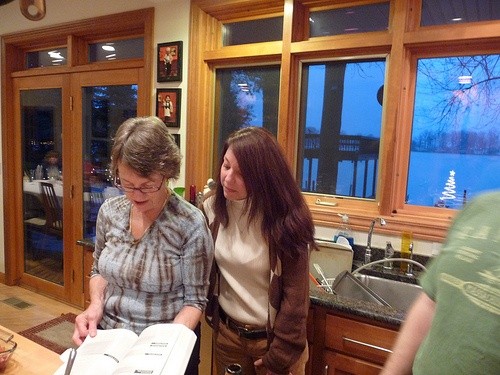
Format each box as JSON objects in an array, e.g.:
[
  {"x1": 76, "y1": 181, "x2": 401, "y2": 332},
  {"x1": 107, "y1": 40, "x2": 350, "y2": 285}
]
[
  {"x1": 86, "y1": 188, "x2": 106, "y2": 233},
  {"x1": 40, "y1": 182, "x2": 64, "y2": 230}
]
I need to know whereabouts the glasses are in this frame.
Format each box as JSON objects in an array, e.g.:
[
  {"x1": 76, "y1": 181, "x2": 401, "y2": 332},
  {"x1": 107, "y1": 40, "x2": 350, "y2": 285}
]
[{"x1": 113, "y1": 168, "x2": 166, "y2": 194}]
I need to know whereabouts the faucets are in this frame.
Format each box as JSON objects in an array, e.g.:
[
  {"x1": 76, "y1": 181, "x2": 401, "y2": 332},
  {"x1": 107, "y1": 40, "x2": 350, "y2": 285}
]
[
  {"x1": 405, "y1": 242, "x2": 414, "y2": 277},
  {"x1": 363, "y1": 217, "x2": 394, "y2": 269}
]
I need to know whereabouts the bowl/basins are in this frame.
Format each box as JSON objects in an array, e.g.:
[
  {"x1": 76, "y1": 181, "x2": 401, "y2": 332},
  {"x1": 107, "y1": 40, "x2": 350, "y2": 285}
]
[{"x1": 0, "y1": 338, "x2": 18, "y2": 370}]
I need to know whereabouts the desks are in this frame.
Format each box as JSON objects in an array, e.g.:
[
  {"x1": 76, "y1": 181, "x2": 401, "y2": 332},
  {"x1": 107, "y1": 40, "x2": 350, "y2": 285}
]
[{"x1": 23, "y1": 178, "x2": 108, "y2": 220}]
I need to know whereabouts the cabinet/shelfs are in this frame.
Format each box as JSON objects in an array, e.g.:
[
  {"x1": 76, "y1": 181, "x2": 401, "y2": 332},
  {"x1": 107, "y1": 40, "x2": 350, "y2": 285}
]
[{"x1": 306, "y1": 302, "x2": 402, "y2": 375}]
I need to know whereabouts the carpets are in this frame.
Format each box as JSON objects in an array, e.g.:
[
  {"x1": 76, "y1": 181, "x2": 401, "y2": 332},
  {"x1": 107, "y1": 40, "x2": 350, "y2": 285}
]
[{"x1": 17, "y1": 313, "x2": 78, "y2": 355}]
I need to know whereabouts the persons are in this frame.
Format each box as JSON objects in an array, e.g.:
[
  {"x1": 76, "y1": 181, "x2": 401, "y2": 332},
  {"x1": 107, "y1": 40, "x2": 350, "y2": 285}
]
[
  {"x1": 161, "y1": 95, "x2": 173, "y2": 123},
  {"x1": 36, "y1": 151, "x2": 60, "y2": 179},
  {"x1": 73, "y1": 117, "x2": 216, "y2": 375},
  {"x1": 84, "y1": 150, "x2": 97, "y2": 180},
  {"x1": 200, "y1": 128, "x2": 321, "y2": 375},
  {"x1": 163, "y1": 46, "x2": 173, "y2": 77},
  {"x1": 380, "y1": 190, "x2": 500, "y2": 375},
  {"x1": 128, "y1": 188, "x2": 171, "y2": 244}
]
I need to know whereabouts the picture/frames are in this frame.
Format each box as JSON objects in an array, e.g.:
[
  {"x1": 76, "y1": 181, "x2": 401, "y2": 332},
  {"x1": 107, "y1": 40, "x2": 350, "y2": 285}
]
[
  {"x1": 157, "y1": 41, "x2": 183, "y2": 83},
  {"x1": 155, "y1": 88, "x2": 182, "y2": 128}
]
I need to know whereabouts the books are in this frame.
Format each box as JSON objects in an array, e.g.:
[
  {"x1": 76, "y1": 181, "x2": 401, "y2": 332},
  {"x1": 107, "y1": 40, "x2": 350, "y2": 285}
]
[{"x1": 69, "y1": 323, "x2": 198, "y2": 375}]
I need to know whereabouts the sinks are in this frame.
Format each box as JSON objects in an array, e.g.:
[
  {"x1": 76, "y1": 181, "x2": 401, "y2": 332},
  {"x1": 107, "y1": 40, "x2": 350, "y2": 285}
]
[
  {"x1": 308, "y1": 264, "x2": 357, "y2": 294},
  {"x1": 331, "y1": 274, "x2": 422, "y2": 310}
]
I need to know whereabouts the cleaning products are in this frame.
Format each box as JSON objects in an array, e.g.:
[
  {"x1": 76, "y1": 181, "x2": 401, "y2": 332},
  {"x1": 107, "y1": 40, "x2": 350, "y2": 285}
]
[{"x1": 334, "y1": 214, "x2": 354, "y2": 262}]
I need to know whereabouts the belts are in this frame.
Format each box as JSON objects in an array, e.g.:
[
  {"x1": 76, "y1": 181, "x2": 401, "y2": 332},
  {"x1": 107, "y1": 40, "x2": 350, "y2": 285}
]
[{"x1": 218, "y1": 306, "x2": 268, "y2": 340}]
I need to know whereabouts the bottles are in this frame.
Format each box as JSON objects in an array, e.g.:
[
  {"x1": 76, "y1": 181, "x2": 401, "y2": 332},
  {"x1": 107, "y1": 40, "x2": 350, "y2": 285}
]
[
  {"x1": 90, "y1": 169, "x2": 112, "y2": 188},
  {"x1": 333, "y1": 213, "x2": 353, "y2": 248},
  {"x1": 188, "y1": 184, "x2": 197, "y2": 206},
  {"x1": 400, "y1": 230, "x2": 412, "y2": 274}
]
